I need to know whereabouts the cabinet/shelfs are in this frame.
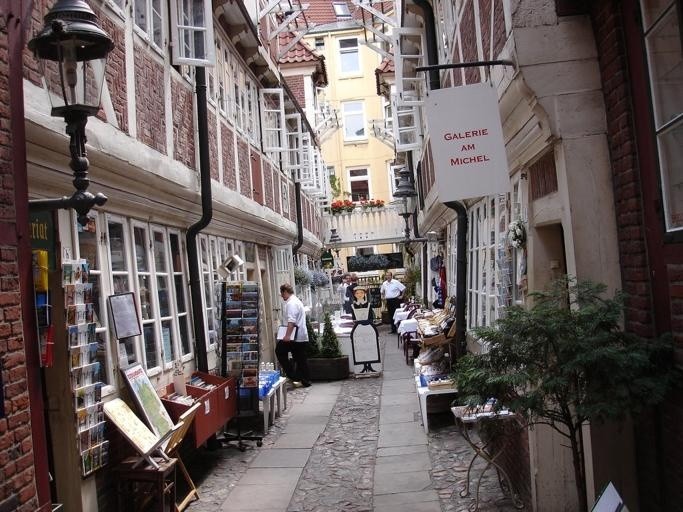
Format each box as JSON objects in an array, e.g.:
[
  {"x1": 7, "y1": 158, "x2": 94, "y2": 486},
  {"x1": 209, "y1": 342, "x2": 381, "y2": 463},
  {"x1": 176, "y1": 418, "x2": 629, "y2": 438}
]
[{"x1": 219, "y1": 279, "x2": 262, "y2": 452}]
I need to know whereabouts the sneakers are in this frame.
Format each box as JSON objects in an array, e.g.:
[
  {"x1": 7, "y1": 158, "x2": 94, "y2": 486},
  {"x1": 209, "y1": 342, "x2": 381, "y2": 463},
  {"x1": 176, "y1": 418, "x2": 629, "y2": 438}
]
[{"x1": 283, "y1": 375, "x2": 311, "y2": 387}]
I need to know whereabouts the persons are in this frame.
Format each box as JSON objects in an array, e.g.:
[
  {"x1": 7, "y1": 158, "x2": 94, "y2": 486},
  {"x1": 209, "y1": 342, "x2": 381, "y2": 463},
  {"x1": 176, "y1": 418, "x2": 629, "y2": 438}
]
[
  {"x1": 380, "y1": 271, "x2": 407, "y2": 334},
  {"x1": 350, "y1": 286, "x2": 382, "y2": 374},
  {"x1": 345, "y1": 273, "x2": 360, "y2": 325},
  {"x1": 274, "y1": 283, "x2": 311, "y2": 388},
  {"x1": 336, "y1": 273, "x2": 352, "y2": 314}
]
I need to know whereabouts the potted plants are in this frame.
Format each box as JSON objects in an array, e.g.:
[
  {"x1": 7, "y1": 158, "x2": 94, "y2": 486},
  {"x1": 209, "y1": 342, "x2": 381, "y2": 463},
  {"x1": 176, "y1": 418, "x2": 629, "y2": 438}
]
[{"x1": 304, "y1": 312, "x2": 350, "y2": 384}]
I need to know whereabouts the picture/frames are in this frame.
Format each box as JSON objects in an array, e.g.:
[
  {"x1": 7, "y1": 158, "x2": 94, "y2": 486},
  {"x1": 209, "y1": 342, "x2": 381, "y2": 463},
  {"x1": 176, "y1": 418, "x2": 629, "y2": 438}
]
[{"x1": 109, "y1": 291, "x2": 143, "y2": 339}]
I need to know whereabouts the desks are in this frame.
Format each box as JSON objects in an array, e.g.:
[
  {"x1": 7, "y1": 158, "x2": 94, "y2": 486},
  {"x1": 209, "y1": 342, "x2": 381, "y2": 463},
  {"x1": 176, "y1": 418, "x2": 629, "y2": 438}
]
[
  {"x1": 413, "y1": 356, "x2": 458, "y2": 435},
  {"x1": 451, "y1": 398, "x2": 525, "y2": 511}
]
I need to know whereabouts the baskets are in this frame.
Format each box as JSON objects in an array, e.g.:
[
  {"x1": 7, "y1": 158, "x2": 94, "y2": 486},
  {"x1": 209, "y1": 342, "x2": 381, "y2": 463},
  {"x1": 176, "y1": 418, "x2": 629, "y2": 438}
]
[
  {"x1": 446, "y1": 318, "x2": 457, "y2": 337},
  {"x1": 421, "y1": 333, "x2": 446, "y2": 346}
]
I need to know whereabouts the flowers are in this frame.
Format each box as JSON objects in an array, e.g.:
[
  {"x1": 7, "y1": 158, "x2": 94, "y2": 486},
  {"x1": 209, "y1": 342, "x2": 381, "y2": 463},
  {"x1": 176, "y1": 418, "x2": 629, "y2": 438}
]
[
  {"x1": 506, "y1": 221, "x2": 525, "y2": 249},
  {"x1": 330, "y1": 198, "x2": 384, "y2": 213}
]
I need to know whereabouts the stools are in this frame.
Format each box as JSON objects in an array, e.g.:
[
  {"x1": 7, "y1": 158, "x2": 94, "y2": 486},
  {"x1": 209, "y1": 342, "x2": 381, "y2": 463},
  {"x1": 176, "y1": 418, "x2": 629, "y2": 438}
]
[
  {"x1": 121, "y1": 454, "x2": 180, "y2": 511},
  {"x1": 254, "y1": 377, "x2": 288, "y2": 435}
]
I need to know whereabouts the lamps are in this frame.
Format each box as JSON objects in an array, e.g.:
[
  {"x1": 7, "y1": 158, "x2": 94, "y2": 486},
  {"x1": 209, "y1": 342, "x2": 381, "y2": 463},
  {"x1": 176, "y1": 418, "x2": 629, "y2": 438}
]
[
  {"x1": 391, "y1": 163, "x2": 444, "y2": 246},
  {"x1": 329, "y1": 228, "x2": 343, "y2": 258},
  {"x1": 12, "y1": 0, "x2": 112, "y2": 223}
]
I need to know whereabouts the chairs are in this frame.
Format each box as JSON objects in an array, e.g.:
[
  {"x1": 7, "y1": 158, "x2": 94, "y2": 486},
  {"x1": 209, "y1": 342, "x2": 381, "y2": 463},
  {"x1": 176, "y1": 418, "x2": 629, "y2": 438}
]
[{"x1": 394, "y1": 301, "x2": 430, "y2": 363}]
